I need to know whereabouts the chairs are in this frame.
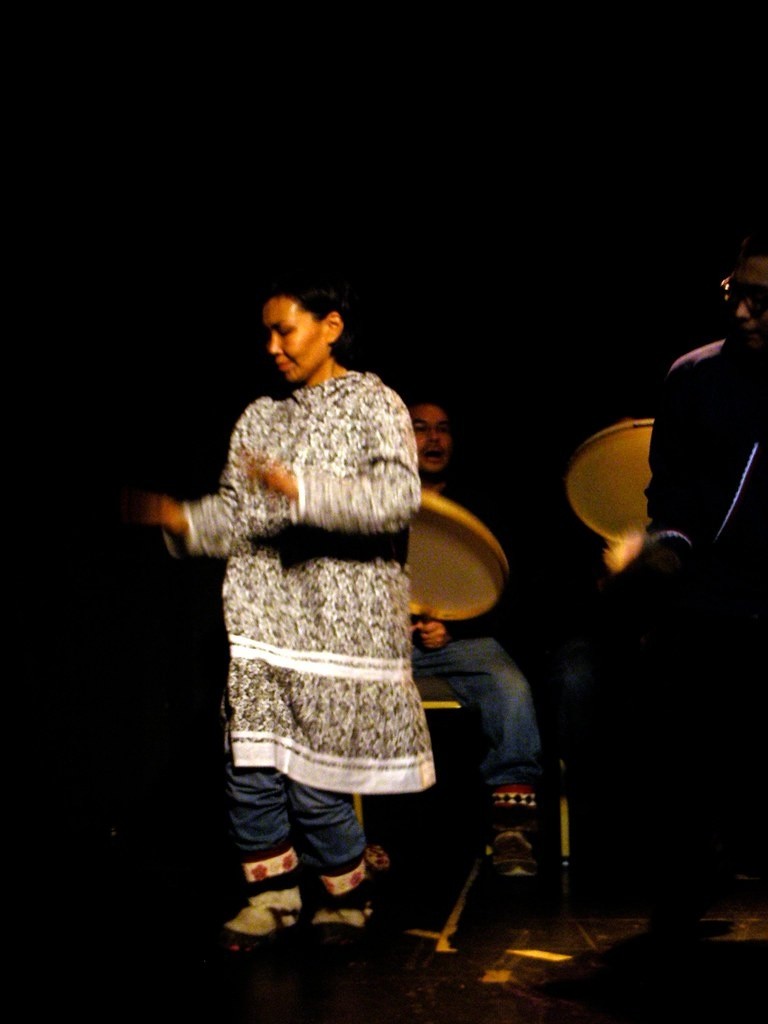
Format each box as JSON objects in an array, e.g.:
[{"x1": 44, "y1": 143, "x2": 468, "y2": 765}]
[{"x1": 350, "y1": 676, "x2": 600, "y2": 954}]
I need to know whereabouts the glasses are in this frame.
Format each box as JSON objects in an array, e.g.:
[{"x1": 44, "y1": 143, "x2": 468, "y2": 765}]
[{"x1": 725, "y1": 281, "x2": 768, "y2": 316}]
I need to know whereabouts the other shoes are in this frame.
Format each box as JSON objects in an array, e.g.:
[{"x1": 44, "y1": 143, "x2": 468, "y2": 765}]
[
  {"x1": 490, "y1": 791, "x2": 541, "y2": 877},
  {"x1": 218, "y1": 843, "x2": 306, "y2": 951},
  {"x1": 310, "y1": 859, "x2": 373, "y2": 945}
]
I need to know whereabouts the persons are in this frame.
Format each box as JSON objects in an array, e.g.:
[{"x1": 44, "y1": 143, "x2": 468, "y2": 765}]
[
  {"x1": 603, "y1": 235, "x2": 767, "y2": 977},
  {"x1": 138, "y1": 265, "x2": 437, "y2": 972},
  {"x1": 363, "y1": 402, "x2": 542, "y2": 879}
]
